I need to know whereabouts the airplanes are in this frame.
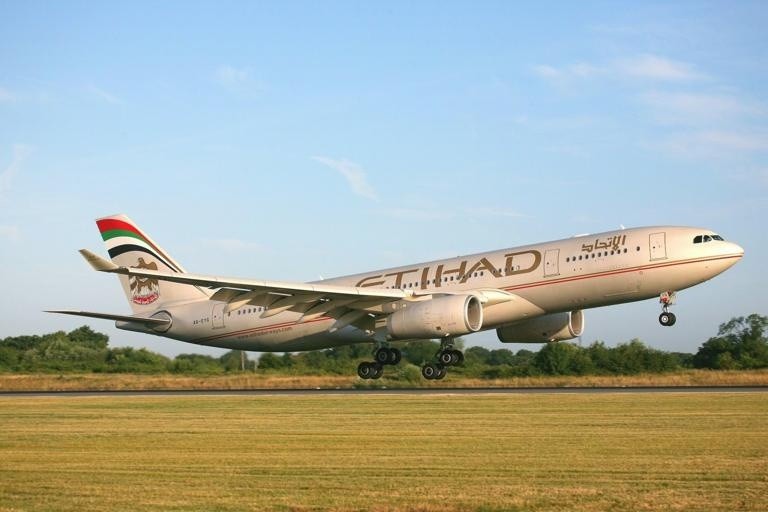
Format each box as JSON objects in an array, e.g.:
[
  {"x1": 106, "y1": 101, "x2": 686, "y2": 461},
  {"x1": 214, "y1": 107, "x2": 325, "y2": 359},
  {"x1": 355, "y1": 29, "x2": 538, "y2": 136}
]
[{"x1": 39, "y1": 198, "x2": 746, "y2": 383}]
